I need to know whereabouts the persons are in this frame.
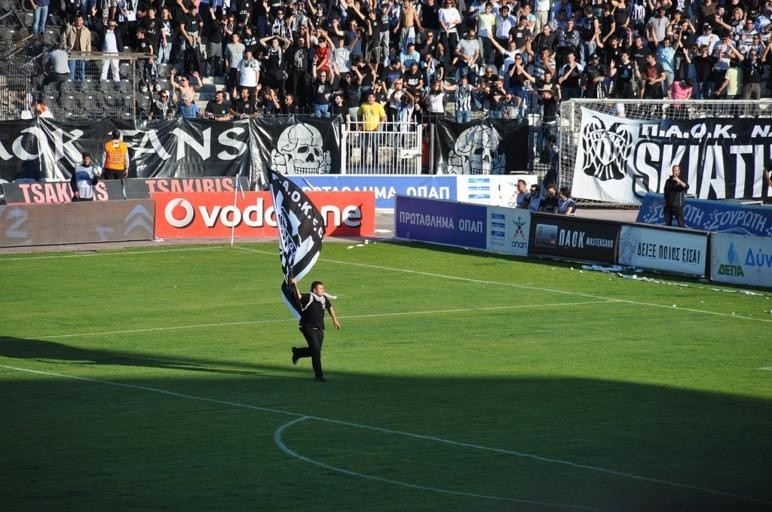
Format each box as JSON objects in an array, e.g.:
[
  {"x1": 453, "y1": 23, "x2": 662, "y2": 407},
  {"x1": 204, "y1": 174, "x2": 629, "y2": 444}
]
[
  {"x1": 662, "y1": 164, "x2": 690, "y2": 229},
  {"x1": 542, "y1": 183, "x2": 561, "y2": 215},
  {"x1": 526, "y1": 184, "x2": 545, "y2": 213},
  {"x1": 288, "y1": 277, "x2": 342, "y2": 382},
  {"x1": 515, "y1": 179, "x2": 531, "y2": 209},
  {"x1": 101, "y1": 129, "x2": 130, "y2": 181},
  {"x1": 69, "y1": 152, "x2": 100, "y2": 202},
  {"x1": 556, "y1": 187, "x2": 576, "y2": 218}
]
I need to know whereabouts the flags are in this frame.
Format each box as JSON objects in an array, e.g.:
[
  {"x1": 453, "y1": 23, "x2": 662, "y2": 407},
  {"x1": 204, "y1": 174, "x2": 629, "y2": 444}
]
[{"x1": 265, "y1": 163, "x2": 326, "y2": 324}]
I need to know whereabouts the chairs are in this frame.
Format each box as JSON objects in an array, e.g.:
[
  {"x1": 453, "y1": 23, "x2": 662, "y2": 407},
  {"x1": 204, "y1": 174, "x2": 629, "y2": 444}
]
[{"x1": 0, "y1": 1, "x2": 161, "y2": 122}]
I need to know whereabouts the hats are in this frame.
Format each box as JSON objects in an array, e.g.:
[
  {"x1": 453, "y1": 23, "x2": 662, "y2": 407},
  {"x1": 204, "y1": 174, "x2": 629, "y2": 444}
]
[{"x1": 538, "y1": 84, "x2": 553, "y2": 94}]
